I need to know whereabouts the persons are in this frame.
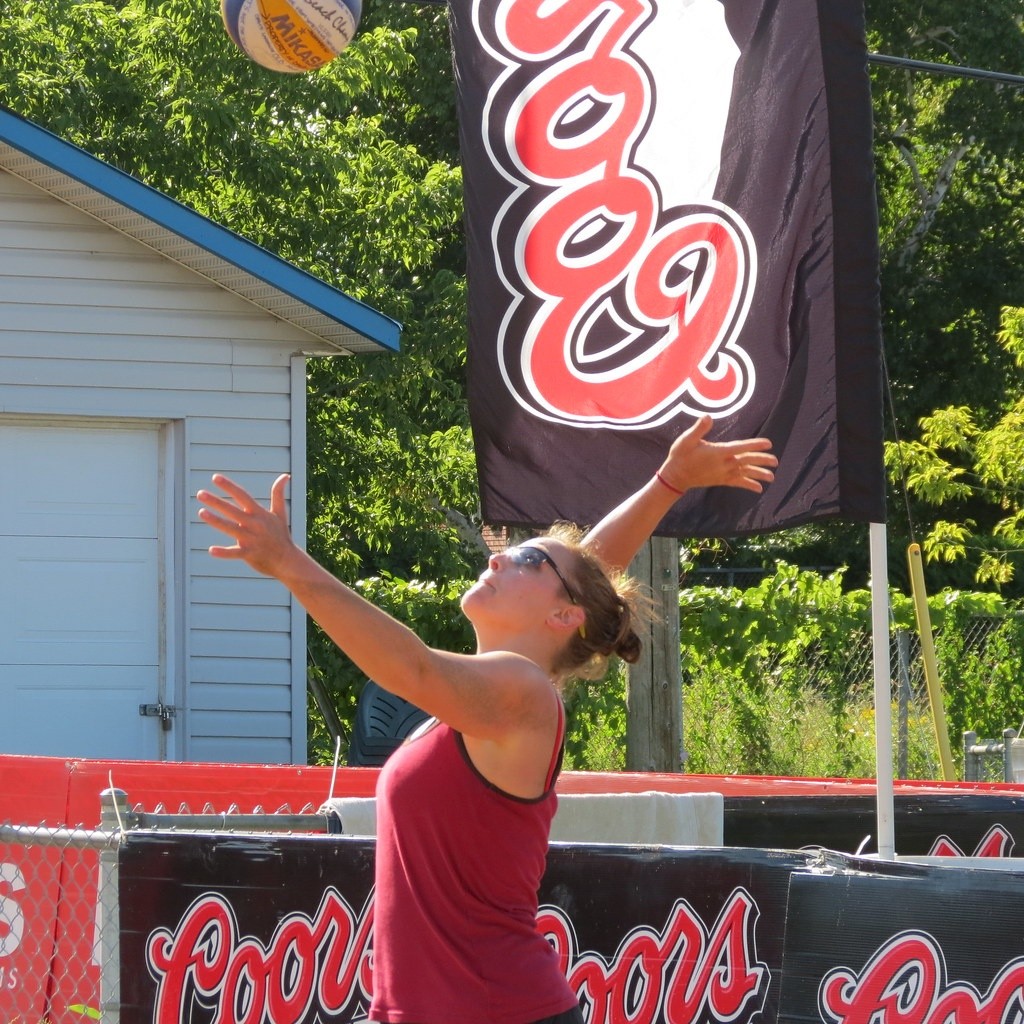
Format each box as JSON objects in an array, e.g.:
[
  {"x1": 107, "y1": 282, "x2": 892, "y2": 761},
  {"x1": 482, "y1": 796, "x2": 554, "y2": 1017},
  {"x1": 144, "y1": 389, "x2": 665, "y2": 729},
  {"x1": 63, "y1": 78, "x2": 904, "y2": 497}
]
[{"x1": 196, "y1": 413, "x2": 778, "y2": 1024}]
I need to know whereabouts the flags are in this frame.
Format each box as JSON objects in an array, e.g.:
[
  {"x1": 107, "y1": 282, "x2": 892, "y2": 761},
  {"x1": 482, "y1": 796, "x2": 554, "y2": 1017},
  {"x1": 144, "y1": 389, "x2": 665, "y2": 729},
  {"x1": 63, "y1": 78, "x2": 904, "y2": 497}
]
[{"x1": 450, "y1": 0, "x2": 885, "y2": 537}]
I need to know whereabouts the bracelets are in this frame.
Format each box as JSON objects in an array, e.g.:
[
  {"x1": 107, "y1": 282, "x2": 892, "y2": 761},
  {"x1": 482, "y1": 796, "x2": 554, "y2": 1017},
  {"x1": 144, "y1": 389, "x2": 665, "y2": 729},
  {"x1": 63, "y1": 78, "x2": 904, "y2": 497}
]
[{"x1": 655, "y1": 471, "x2": 685, "y2": 494}]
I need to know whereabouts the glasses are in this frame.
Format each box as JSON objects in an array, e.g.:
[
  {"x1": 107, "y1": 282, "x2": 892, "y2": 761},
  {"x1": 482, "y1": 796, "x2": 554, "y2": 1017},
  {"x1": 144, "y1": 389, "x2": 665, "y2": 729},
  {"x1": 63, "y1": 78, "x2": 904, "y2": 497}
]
[{"x1": 503, "y1": 546, "x2": 586, "y2": 639}]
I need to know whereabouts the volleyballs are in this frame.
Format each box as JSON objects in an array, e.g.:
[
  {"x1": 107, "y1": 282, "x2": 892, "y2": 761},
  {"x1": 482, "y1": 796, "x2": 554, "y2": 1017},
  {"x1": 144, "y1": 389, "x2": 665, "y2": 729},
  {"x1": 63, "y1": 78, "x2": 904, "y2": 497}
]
[{"x1": 219, "y1": 0, "x2": 362, "y2": 74}]
